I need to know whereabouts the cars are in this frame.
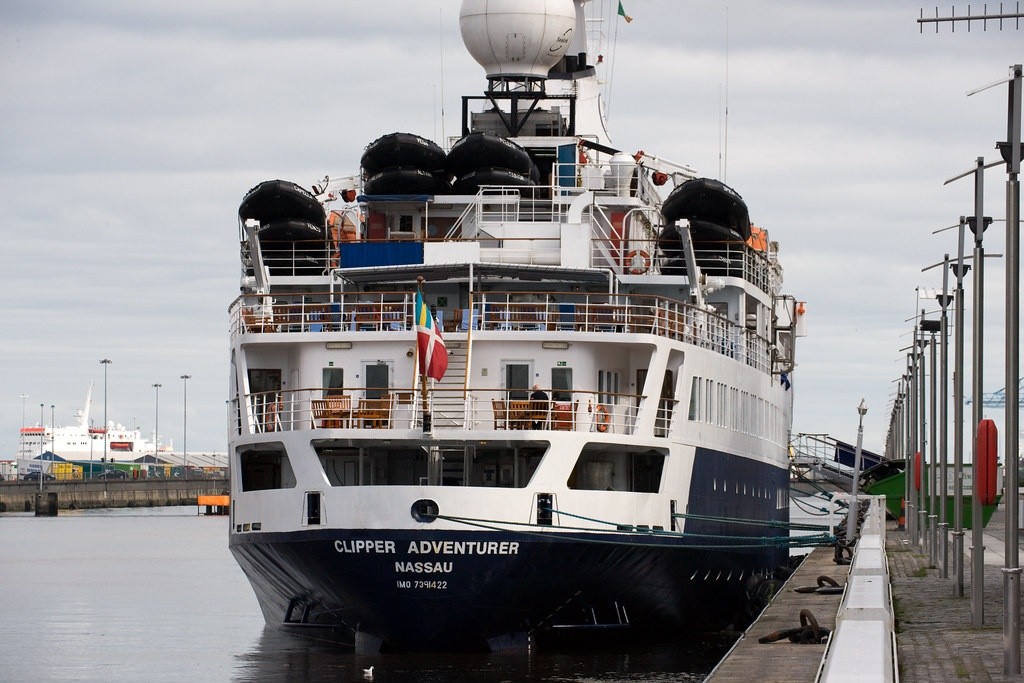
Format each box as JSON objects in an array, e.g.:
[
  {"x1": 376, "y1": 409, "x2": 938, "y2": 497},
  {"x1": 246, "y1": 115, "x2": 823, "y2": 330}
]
[
  {"x1": 24, "y1": 471, "x2": 56, "y2": 482},
  {"x1": 97, "y1": 469, "x2": 130, "y2": 480}
]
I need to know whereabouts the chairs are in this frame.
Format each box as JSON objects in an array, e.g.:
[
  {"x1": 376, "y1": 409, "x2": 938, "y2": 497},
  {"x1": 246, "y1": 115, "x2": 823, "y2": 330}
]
[
  {"x1": 312, "y1": 394, "x2": 393, "y2": 429},
  {"x1": 492, "y1": 398, "x2": 579, "y2": 430}
]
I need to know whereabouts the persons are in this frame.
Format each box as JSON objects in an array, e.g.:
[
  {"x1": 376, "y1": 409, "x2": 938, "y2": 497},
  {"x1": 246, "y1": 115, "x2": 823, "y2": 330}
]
[{"x1": 526, "y1": 385, "x2": 550, "y2": 430}]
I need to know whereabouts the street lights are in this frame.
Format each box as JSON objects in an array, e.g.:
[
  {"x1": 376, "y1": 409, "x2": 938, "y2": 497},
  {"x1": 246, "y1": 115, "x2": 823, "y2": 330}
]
[
  {"x1": 99, "y1": 358, "x2": 113, "y2": 480},
  {"x1": 180, "y1": 375, "x2": 192, "y2": 466},
  {"x1": 50, "y1": 404, "x2": 55, "y2": 476},
  {"x1": 39, "y1": 403, "x2": 45, "y2": 492},
  {"x1": 845, "y1": 396, "x2": 868, "y2": 547},
  {"x1": 151, "y1": 383, "x2": 164, "y2": 464}
]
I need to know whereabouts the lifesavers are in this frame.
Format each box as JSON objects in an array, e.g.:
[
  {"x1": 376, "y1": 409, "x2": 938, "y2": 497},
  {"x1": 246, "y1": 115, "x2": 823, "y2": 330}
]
[
  {"x1": 596, "y1": 405, "x2": 609, "y2": 433},
  {"x1": 265, "y1": 404, "x2": 276, "y2": 431},
  {"x1": 626, "y1": 249, "x2": 651, "y2": 275}
]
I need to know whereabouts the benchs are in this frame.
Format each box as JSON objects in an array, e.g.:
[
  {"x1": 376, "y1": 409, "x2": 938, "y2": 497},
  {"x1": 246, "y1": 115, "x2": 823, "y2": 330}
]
[{"x1": 240, "y1": 302, "x2": 703, "y2": 345}]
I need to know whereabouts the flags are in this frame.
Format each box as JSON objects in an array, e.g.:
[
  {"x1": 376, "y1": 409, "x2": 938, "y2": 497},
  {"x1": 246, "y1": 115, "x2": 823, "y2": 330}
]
[
  {"x1": 617, "y1": 0, "x2": 633, "y2": 23},
  {"x1": 415, "y1": 288, "x2": 449, "y2": 381}
]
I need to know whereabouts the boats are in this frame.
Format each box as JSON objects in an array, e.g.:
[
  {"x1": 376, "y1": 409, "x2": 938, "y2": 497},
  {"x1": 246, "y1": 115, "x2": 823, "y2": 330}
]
[
  {"x1": 227, "y1": 1, "x2": 809, "y2": 652},
  {"x1": 16, "y1": 379, "x2": 174, "y2": 485}
]
